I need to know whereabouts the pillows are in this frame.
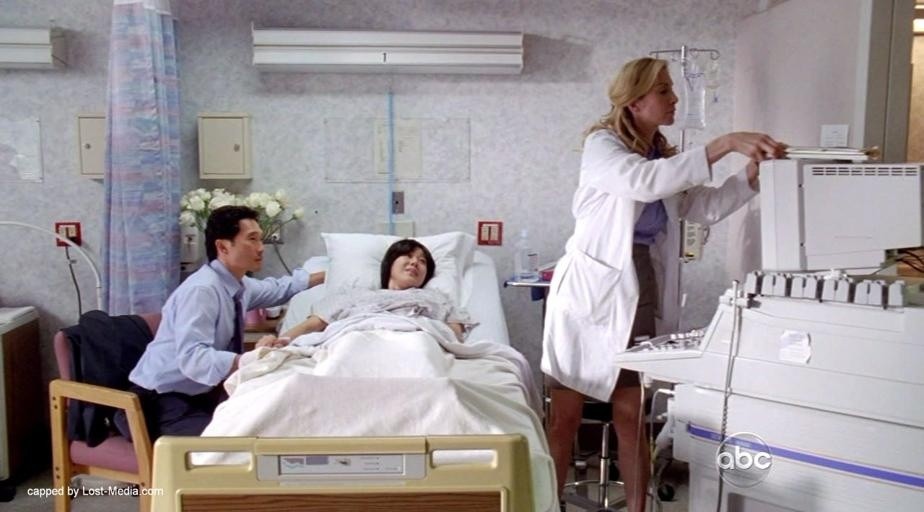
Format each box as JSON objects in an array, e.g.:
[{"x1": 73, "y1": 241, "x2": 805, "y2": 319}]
[{"x1": 320, "y1": 230, "x2": 466, "y2": 303}]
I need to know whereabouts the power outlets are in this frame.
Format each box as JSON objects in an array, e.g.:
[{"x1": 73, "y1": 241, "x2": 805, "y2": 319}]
[{"x1": 58, "y1": 225, "x2": 78, "y2": 240}]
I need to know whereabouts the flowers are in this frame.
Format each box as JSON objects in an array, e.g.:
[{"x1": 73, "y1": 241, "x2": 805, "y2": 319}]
[{"x1": 175, "y1": 188, "x2": 306, "y2": 250}]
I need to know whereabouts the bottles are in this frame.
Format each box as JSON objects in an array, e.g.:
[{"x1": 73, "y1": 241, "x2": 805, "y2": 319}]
[{"x1": 515, "y1": 229, "x2": 539, "y2": 283}]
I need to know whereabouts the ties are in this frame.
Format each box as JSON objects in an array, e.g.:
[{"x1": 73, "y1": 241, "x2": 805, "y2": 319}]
[{"x1": 232, "y1": 294, "x2": 244, "y2": 354}]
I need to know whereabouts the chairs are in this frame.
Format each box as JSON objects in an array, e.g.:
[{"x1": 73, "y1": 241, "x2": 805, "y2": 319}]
[{"x1": 48, "y1": 314, "x2": 166, "y2": 510}]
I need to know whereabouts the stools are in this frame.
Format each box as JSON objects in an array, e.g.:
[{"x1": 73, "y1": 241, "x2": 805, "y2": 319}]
[{"x1": 564, "y1": 403, "x2": 666, "y2": 512}]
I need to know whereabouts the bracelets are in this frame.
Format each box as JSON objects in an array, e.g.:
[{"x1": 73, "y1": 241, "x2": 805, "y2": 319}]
[{"x1": 279, "y1": 336, "x2": 291, "y2": 342}]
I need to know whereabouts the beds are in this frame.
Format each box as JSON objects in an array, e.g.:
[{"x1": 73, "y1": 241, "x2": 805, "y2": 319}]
[{"x1": 152, "y1": 247, "x2": 562, "y2": 512}]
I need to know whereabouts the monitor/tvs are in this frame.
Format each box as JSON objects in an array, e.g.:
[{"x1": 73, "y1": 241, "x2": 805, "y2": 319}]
[{"x1": 760, "y1": 157, "x2": 924, "y2": 275}]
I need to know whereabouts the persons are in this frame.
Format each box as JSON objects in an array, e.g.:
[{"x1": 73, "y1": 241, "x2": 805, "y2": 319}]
[
  {"x1": 542, "y1": 58, "x2": 791, "y2": 510},
  {"x1": 250, "y1": 237, "x2": 463, "y2": 355},
  {"x1": 126, "y1": 205, "x2": 325, "y2": 437}
]
